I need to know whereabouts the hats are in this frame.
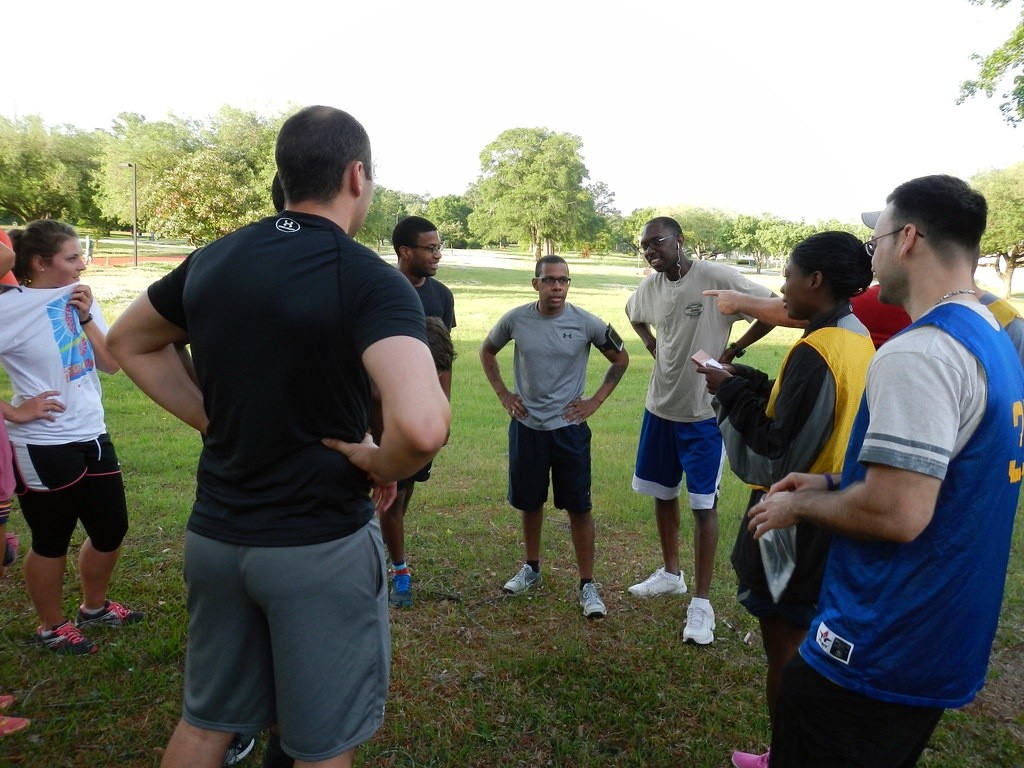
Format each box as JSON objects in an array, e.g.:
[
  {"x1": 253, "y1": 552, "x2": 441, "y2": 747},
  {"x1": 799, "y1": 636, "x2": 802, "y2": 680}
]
[{"x1": 861, "y1": 210, "x2": 884, "y2": 230}]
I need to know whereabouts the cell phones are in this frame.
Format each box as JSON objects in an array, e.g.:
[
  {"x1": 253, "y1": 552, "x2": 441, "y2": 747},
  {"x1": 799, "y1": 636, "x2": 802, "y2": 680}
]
[{"x1": 606, "y1": 325, "x2": 625, "y2": 351}]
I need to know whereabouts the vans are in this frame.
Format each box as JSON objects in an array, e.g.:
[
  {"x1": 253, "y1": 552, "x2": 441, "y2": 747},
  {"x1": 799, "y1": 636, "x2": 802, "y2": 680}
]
[{"x1": 736, "y1": 256, "x2": 758, "y2": 265}]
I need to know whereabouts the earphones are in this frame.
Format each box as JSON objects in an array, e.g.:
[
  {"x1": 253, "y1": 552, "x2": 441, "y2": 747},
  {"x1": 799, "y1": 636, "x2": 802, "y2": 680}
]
[{"x1": 677, "y1": 242, "x2": 679, "y2": 249}]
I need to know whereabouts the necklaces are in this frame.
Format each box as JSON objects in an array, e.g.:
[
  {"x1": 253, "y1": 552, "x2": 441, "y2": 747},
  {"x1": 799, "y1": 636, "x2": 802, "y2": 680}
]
[{"x1": 933, "y1": 289, "x2": 975, "y2": 306}]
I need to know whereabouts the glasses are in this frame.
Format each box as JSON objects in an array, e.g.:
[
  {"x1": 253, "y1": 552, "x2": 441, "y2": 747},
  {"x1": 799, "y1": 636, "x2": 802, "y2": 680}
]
[
  {"x1": 638, "y1": 234, "x2": 683, "y2": 255},
  {"x1": 536, "y1": 277, "x2": 571, "y2": 286},
  {"x1": 862, "y1": 227, "x2": 924, "y2": 256},
  {"x1": 407, "y1": 244, "x2": 442, "y2": 254}
]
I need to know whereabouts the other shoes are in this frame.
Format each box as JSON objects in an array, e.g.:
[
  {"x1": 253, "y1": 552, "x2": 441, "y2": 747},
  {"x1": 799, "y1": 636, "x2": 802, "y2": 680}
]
[
  {"x1": 3, "y1": 544, "x2": 15, "y2": 567},
  {"x1": 0, "y1": 695, "x2": 16, "y2": 709},
  {"x1": 0, "y1": 714, "x2": 30, "y2": 737}
]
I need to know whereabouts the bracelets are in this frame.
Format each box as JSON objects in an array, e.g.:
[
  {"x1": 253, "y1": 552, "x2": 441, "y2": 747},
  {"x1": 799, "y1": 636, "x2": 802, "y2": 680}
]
[
  {"x1": 80, "y1": 313, "x2": 93, "y2": 325},
  {"x1": 825, "y1": 473, "x2": 835, "y2": 490}
]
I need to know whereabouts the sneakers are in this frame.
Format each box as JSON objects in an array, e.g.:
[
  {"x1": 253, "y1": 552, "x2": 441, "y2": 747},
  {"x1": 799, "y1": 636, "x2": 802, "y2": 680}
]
[
  {"x1": 223, "y1": 732, "x2": 256, "y2": 767},
  {"x1": 389, "y1": 574, "x2": 413, "y2": 607},
  {"x1": 503, "y1": 564, "x2": 540, "y2": 594},
  {"x1": 37, "y1": 618, "x2": 97, "y2": 656},
  {"x1": 683, "y1": 597, "x2": 716, "y2": 645},
  {"x1": 74, "y1": 599, "x2": 145, "y2": 628},
  {"x1": 580, "y1": 580, "x2": 607, "y2": 618},
  {"x1": 627, "y1": 567, "x2": 687, "y2": 598},
  {"x1": 732, "y1": 748, "x2": 770, "y2": 768}
]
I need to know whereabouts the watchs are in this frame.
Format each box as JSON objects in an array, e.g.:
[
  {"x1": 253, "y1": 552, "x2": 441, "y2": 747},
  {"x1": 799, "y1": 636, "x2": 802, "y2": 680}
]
[{"x1": 731, "y1": 343, "x2": 746, "y2": 358}]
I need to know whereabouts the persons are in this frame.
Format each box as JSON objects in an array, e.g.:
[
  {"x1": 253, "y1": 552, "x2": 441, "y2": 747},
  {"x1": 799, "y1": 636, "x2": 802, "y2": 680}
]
[
  {"x1": 624, "y1": 217, "x2": 779, "y2": 647},
  {"x1": 698, "y1": 231, "x2": 874, "y2": 768},
  {"x1": 106, "y1": 106, "x2": 452, "y2": 767},
  {"x1": 379, "y1": 216, "x2": 457, "y2": 607},
  {"x1": 0, "y1": 220, "x2": 145, "y2": 734},
  {"x1": 747, "y1": 176, "x2": 1024, "y2": 768},
  {"x1": 703, "y1": 212, "x2": 1023, "y2": 363},
  {"x1": 479, "y1": 256, "x2": 629, "y2": 617}
]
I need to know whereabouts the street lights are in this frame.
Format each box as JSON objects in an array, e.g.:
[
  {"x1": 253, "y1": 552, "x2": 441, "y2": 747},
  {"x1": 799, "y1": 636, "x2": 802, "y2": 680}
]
[{"x1": 126, "y1": 162, "x2": 138, "y2": 267}]
